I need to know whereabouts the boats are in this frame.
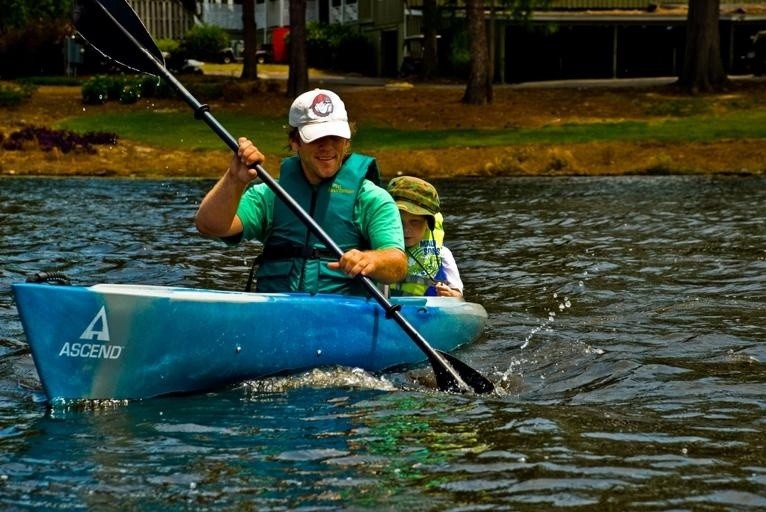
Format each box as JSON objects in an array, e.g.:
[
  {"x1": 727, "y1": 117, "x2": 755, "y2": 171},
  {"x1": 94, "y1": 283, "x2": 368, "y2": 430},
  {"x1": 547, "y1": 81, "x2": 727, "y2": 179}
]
[{"x1": 8, "y1": 269, "x2": 490, "y2": 407}]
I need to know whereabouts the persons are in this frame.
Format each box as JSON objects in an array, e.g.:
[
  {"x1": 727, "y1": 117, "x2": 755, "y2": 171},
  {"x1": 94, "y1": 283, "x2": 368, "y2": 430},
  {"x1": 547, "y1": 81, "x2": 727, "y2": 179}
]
[
  {"x1": 195, "y1": 85, "x2": 407, "y2": 298},
  {"x1": 384, "y1": 175, "x2": 467, "y2": 300}
]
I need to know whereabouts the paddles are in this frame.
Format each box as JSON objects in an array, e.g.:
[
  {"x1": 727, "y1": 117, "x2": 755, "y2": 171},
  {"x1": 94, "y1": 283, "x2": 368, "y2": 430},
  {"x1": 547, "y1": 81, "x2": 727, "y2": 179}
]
[{"x1": 72, "y1": 0, "x2": 494, "y2": 394}]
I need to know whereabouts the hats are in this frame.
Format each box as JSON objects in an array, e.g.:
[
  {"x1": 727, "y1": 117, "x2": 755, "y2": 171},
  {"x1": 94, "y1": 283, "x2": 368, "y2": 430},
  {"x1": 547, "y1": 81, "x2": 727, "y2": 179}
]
[
  {"x1": 289, "y1": 88, "x2": 352, "y2": 143},
  {"x1": 387, "y1": 176, "x2": 440, "y2": 215}
]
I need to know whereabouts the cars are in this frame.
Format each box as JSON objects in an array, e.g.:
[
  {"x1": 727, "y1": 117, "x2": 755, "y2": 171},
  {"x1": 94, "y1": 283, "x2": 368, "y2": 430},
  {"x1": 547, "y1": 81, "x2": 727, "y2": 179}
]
[{"x1": 218, "y1": 38, "x2": 266, "y2": 66}]
